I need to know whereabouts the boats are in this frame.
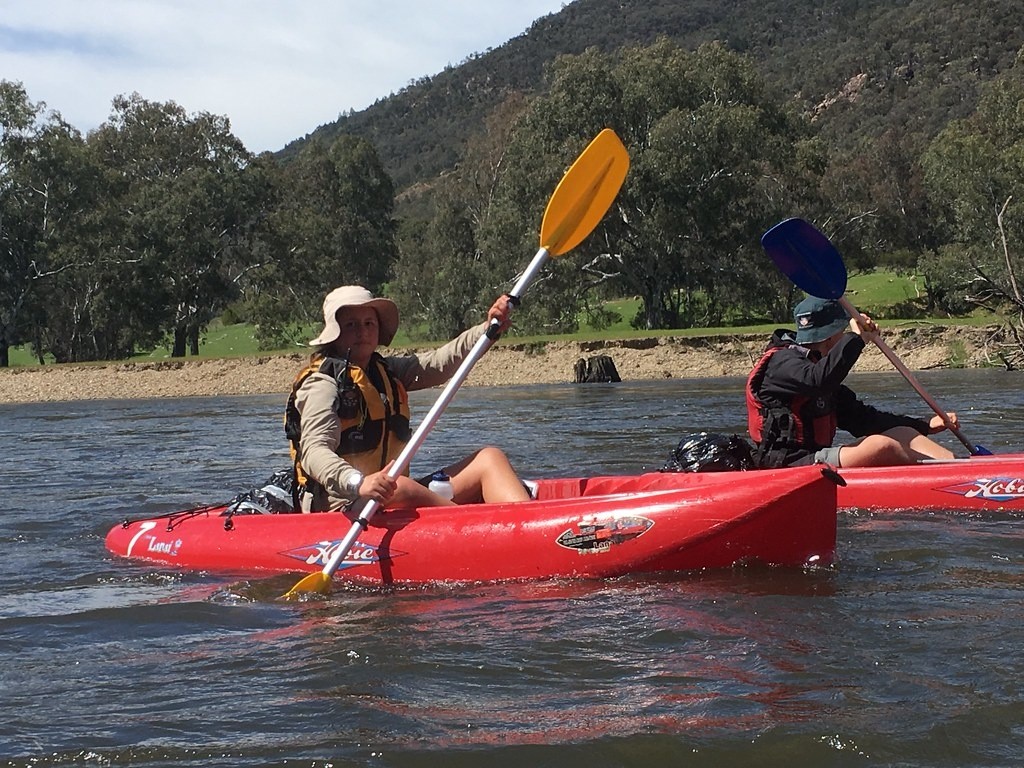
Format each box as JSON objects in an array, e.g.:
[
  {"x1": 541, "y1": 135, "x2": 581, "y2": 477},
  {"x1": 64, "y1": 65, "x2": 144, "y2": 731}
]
[
  {"x1": 109, "y1": 463, "x2": 845, "y2": 581},
  {"x1": 654, "y1": 432, "x2": 1024, "y2": 512}
]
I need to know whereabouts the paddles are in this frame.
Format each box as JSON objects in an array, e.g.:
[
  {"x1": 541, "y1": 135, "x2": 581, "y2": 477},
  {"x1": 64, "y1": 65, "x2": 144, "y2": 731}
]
[
  {"x1": 277, "y1": 127, "x2": 630, "y2": 602},
  {"x1": 760, "y1": 218, "x2": 995, "y2": 456}
]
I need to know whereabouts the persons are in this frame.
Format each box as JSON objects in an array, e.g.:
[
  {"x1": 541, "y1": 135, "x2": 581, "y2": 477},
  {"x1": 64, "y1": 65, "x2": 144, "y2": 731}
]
[
  {"x1": 745, "y1": 298, "x2": 961, "y2": 467},
  {"x1": 284, "y1": 285, "x2": 533, "y2": 509}
]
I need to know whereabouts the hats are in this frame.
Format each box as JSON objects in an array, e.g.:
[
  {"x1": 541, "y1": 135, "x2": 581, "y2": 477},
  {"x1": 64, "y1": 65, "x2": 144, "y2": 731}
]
[
  {"x1": 307, "y1": 284, "x2": 403, "y2": 351},
  {"x1": 793, "y1": 298, "x2": 853, "y2": 345}
]
